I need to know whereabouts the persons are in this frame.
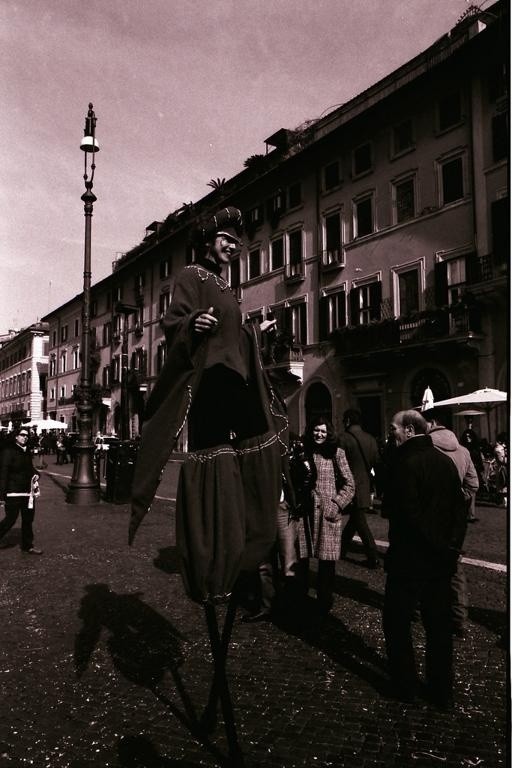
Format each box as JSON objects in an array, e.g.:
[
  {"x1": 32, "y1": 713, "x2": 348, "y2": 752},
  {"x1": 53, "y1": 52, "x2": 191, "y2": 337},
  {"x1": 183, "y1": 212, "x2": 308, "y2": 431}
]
[
  {"x1": 272, "y1": 406, "x2": 506, "y2": 712},
  {"x1": 128, "y1": 206, "x2": 287, "y2": 608},
  {"x1": 1, "y1": 427, "x2": 44, "y2": 554},
  {"x1": 30, "y1": 426, "x2": 72, "y2": 465}
]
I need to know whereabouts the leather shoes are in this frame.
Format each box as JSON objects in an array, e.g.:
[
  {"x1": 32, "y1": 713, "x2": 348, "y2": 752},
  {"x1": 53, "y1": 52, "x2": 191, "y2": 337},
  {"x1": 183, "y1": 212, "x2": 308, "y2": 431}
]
[{"x1": 240, "y1": 594, "x2": 275, "y2": 623}]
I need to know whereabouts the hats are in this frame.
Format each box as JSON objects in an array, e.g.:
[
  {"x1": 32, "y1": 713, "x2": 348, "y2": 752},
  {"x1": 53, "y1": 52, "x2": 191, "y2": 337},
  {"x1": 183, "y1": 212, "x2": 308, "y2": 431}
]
[{"x1": 192, "y1": 206, "x2": 244, "y2": 261}]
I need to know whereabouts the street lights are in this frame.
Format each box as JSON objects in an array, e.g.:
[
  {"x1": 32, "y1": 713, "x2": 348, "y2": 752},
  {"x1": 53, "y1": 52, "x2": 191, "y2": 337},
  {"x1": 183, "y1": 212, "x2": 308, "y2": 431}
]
[
  {"x1": 112, "y1": 272, "x2": 141, "y2": 441},
  {"x1": 61, "y1": 102, "x2": 98, "y2": 503}
]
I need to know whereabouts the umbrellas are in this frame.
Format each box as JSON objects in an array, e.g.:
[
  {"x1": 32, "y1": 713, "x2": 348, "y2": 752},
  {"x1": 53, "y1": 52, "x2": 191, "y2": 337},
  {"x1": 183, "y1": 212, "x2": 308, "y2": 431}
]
[{"x1": 429, "y1": 383, "x2": 508, "y2": 441}]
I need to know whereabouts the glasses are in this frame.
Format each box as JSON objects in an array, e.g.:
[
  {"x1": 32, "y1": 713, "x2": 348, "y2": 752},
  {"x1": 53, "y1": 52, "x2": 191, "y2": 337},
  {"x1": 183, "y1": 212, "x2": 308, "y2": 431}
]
[{"x1": 18, "y1": 434, "x2": 29, "y2": 437}]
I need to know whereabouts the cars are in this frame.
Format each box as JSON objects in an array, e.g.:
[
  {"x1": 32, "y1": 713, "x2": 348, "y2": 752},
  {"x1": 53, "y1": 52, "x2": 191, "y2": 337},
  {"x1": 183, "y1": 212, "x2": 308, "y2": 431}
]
[{"x1": 93, "y1": 434, "x2": 127, "y2": 458}]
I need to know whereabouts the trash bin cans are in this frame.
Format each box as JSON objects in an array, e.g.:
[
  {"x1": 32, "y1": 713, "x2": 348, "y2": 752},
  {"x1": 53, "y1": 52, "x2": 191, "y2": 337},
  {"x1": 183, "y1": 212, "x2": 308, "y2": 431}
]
[{"x1": 98, "y1": 440, "x2": 137, "y2": 502}]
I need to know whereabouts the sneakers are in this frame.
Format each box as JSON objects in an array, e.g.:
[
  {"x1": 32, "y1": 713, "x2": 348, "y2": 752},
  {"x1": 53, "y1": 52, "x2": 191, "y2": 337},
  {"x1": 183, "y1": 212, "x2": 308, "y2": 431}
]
[{"x1": 23, "y1": 548, "x2": 43, "y2": 554}]
